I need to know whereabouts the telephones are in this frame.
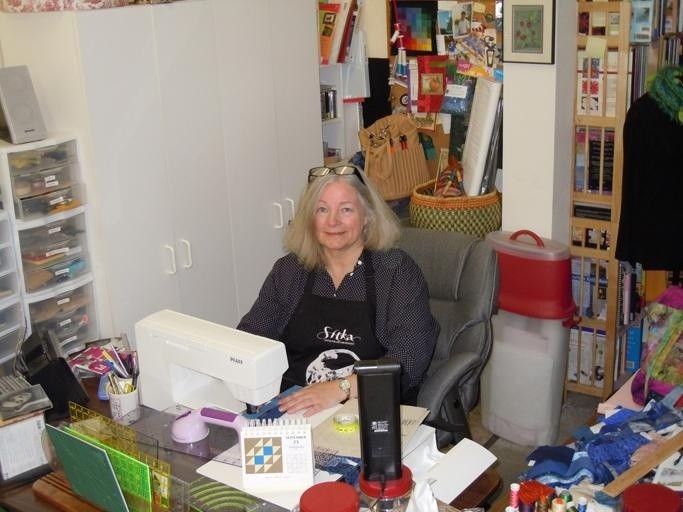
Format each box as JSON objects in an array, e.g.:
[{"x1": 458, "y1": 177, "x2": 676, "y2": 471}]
[{"x1": 353, "y1": 359, "x2": 406, "y2": 481}]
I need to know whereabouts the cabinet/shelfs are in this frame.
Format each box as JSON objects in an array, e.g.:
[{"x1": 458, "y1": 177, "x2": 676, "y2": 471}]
[
  {"x1": 564, "y1": 1, "x2": 682, "y2": 401},
  {"x1": 0, "y1": 0, "x2": 238, "y2": 336},
  {"x1": 0, "y1": 148, "x2": 32, "y2": 367},
  {"x1": 320, "y1": 61, "x2": 362, "y2": 165},
  {"x1": 211, "y1": 0, "x2": 325, "y2": 332},
  {"x1": 1, "y1": 127, "x2": 102, "y2": 359}
]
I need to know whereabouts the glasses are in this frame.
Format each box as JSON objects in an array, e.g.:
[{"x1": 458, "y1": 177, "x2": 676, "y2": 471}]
[{"x1": 306, "y1": 164, "x2": 366, "y2": 187}]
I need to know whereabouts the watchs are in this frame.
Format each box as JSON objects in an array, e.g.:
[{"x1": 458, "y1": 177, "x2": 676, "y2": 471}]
[{"x1": 336, "y1": 376, "x2": 353, "y2": 405}]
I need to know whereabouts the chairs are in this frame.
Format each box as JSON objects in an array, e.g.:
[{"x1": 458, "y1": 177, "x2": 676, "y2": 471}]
[{"x1": 392, "y1": 226, "x2": 497, "y2": 450}]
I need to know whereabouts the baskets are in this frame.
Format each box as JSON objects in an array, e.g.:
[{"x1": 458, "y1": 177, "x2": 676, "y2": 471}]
[{"x1": 408, "y1": 176, "x2": 504, "y2": 238}]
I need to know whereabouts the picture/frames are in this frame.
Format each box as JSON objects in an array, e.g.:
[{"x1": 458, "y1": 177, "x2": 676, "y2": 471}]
[{"x1": 502, "y1": 0, "x2": 555, "y2": 65}]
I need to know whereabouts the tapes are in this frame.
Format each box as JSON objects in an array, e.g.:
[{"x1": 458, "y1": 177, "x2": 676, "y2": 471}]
[{"x1": 334, "y1": 414, "x2": 358, "y2": 432}]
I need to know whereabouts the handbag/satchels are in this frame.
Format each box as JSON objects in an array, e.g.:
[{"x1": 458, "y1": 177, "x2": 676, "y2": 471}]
[{"x1": 358, "y1": 112, "x2": 430, "y2": 201}]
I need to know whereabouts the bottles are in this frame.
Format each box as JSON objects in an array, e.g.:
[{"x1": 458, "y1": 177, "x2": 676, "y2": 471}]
[{"x1": 358, "y1": 464, "x2": 415, "y2": 512}]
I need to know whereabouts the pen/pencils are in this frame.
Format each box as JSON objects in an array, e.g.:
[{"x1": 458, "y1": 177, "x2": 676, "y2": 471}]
[
  {"x1": 443, "y1": 167, "x2": 465, "y2": 198},
  {"x1": 102, "y1": 344, "x2": 128, "y2": 378},
  {"x1": 107, "y1": 373, "x2": 134, "y2": 394},
  {"x1": 388, "y1": 22, "x2": 407, "y2": 81},
  {"x1": 509, "y1": 480, "x2": 588, "y2": 512}
]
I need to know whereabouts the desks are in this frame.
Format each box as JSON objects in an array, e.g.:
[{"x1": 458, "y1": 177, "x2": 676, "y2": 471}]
[
  {"x1": 1, "y1": 371, "x2": 528, "y2": 512},
  {"x1": 485, "y1": 370, "x2": 644, "y2": 512}
]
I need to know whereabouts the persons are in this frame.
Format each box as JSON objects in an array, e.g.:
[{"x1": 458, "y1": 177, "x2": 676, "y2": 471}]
[
  {"x1": 455, "y1": 11, "x2": 471, "y2": 37},
  {"x1": 233, "y1": 163, "x2": 440, "y2": 424},
  {"x1": 1, "y1": 386, "x2": 49, "y2": 413},
  {"x1": 613, "y1": 62, "x2": 683, "y2": 279}
]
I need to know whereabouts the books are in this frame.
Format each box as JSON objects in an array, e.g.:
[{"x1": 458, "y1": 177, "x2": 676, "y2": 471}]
[
  {"x1": 0, "y1": 405, "x2": 52, "y2": 427},
  {"x1": 67, "y1": 344, "x2": 130, "y2": 375},
  {"x1": 0, "y1": 385, "x2": 53, "y2": 422}
]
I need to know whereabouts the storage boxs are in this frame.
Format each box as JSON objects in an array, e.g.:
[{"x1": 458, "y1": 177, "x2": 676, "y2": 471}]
[{"x1": 484, "y1": 230, "x2": 578, "y2": 320}]
